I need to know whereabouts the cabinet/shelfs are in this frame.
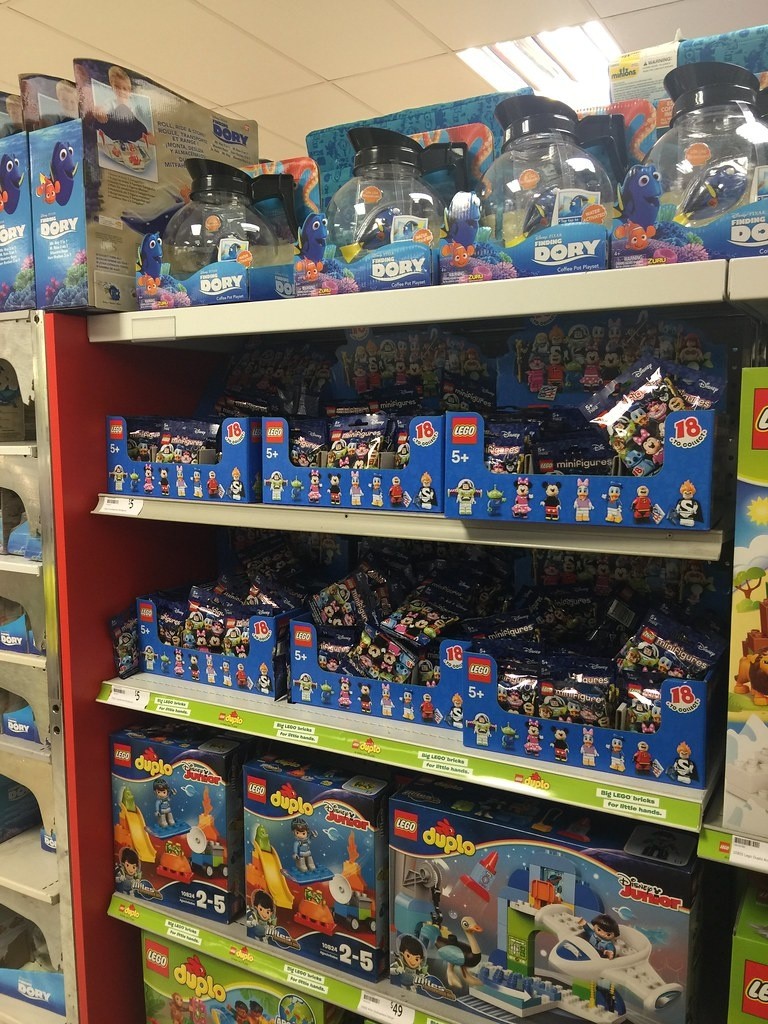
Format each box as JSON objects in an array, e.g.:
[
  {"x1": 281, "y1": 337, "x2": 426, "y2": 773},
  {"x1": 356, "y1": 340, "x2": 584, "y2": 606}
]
[
  {"x1": 1, "y1": 308, "x2": 197, "y2": 1024},
  {"x1": 92, "y1": 256, "x2": 768, "y2": 1024}
]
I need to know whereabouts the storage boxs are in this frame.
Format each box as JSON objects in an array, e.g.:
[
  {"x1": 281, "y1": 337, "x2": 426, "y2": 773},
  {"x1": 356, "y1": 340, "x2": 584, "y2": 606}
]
[
  {"x1": 0, "y1": 601, "x2": 46, "y2": 656},
  {"x1": 0, "y1": 24, "x2": 768, "y2": 311},
  {"x1": 111, "y1": 725, "x2": 727, "y2": 1024},
  {"x1": 100, "y1": 312, "x2": 767, "y2": 533},
  {"x1": 0, "y1": 775, "x2": 57, "y2": 853},
  {"x1": 0, "y1": 928, "x2": 66, "y2": 1017},
  {"x1": 0, "y1": 358, "x2": 36, "y2": 442},
  {"x1": 0, "y1": 488, "x2": 43, "y2": 562},
  {"x1": 726, "y1": 880, "x2": 768, "y2": 1024},
  {"x1": 721, "y1": 364, "x2": 768, "y2": 842},
  {"x1": 138, "y1": 532, "x2": 731, "y2": 788},
  {"x1": 0, "y1": 689, "x2": 41, "y2": 744}
]
